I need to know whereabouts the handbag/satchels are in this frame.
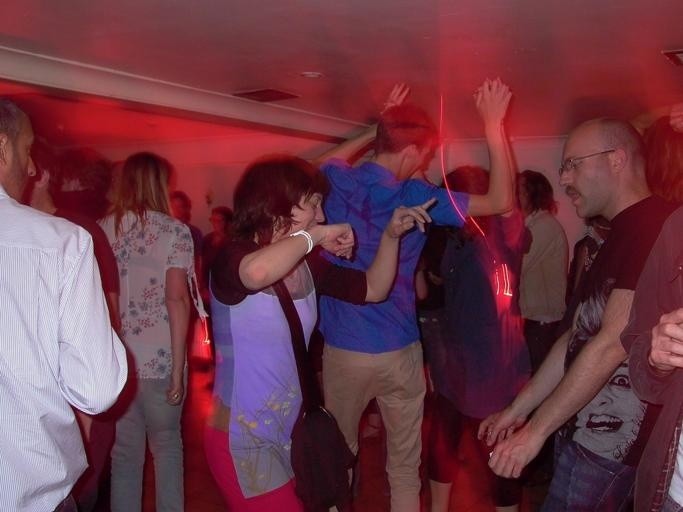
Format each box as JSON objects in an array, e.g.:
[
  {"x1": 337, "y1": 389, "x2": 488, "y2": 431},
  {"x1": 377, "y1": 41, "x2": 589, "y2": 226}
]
[{"x1": 290, "y1": 401, "x2": 353, "y2": 508}]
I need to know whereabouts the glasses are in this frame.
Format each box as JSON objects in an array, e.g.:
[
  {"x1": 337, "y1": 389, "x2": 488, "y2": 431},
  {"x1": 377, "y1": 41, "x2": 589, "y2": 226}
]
[{"x1": 560, "y1": 149, "x2": 615, "y2": 175}]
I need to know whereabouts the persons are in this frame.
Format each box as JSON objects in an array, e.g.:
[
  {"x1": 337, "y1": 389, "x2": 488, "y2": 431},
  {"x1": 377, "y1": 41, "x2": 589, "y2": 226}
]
[{"x1": 0, "y1": 76, "x2": 683, "y2": 510}]
